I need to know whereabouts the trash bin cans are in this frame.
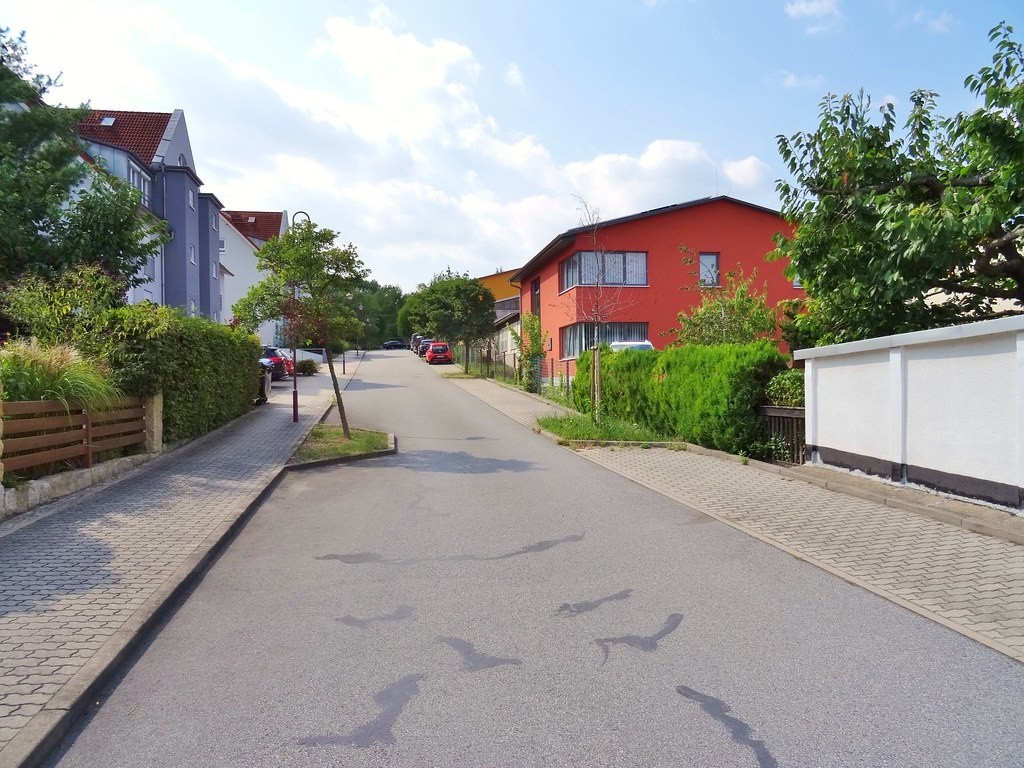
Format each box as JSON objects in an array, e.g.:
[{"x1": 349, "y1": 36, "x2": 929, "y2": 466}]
[{"x1": 253, "y1": 358, "x2": 275, "y2": 406}]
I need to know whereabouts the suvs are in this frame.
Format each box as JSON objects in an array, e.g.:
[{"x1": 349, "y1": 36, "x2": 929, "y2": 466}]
[
  {"x1": 406, "y1": 333, "x2": 436, "y2": 357},
  {"x1": 609, "y1": 339, "x2": 657, "y2": 356},
  {"x1": 260, "y1": 345, "x2": 289, "y2": 380},
  {"x1": 383, "y1": 340, "x2": 404, "y2": 350}
]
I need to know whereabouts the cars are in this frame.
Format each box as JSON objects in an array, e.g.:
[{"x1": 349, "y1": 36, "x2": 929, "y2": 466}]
[
  {"x1": 279, "y1": 349, "x2": 294, "y2": 375},
  {"x1": 426, "y1": 343, "x2": 453, "y2": 364}
]
